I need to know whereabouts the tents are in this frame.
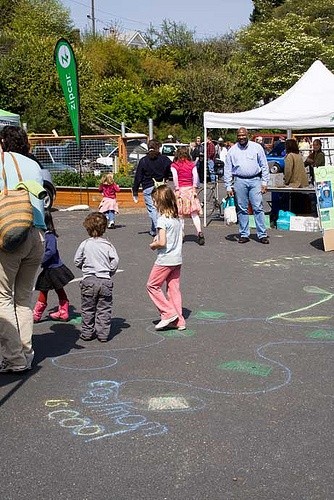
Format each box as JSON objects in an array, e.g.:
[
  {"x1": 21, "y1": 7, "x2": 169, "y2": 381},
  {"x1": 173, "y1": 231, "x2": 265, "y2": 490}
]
[
  {"x1": 204, "y1": 59, "x2": 334, "y2": 229},
  {"x1": 0, "y1": 109, "x2": 21, "y2": 128}
]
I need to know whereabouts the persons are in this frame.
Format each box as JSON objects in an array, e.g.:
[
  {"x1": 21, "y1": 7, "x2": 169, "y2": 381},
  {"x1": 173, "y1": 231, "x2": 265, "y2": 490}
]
[
  {"x1": 285, "y1": 139, "x2": 309, "y2": 187},
  {"x1": 175, "y1": 138, "x2": 181, "y2": 144},
  {"x1": 304, "y1": 139, "x2": 325, "y2": 186},
  {"x1": 206, "y1": 135, "x2": 217, "y2": 183},
  {"x1": 224, "y1": 128, "x2": 270, "y2": 244},
  {"x1": 74, "y1": 211, "x2": 120, "y2": 342},
  {"x1": 171, "y1": 146, "x2": 205, "y2": 245},
  {"x1": 168, "y1": 134, "x2": 174, "y2": 142},
  {"x1": 98, "y1": 174, "x2": 120, "y2": 227},
  {"x1": 32, "y1": 210, "x2": 75, "y2": 322},
  {"x1": 144, "y1": 184, "x2": 187, "y2": 332},
  {"x1": 0, "y1": 126, "x2": 47, "y2": 372},
  {"x1": 194, "y1": 136, "x2": 205, "y2": 182},
  {"x1": 131, "y1": 139, "x2": 174, "y2": 236},
  {"x1": 191, "y1": 142, "x2": 201, "y2": 189}
]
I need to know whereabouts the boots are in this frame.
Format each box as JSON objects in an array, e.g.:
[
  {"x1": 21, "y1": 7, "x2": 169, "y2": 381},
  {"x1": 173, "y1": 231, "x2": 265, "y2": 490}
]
[
  {"x1": 50, "y1": 299, "x2": 69, "y2": 321},
  {"x1": 32, "y1": 301, "x2": 47, "y2": 322}
]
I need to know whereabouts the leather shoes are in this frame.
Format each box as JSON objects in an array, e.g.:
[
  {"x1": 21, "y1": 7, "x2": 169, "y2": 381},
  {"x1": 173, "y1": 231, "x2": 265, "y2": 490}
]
[
  {"x1": 256, "y1": 237, "x2": 269, "y2": 244},
  {"x1": 199, "y1": 232, "x2": 205, "y2": 245},
  {"x1": 240, "y1": 237, "x2": 249, "y2": 243}
]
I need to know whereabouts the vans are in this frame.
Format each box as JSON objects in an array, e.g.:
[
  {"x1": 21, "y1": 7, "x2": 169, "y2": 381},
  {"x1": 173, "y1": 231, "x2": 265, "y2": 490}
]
[{"x1": 252, "y1": 134, "x2": 287, "y2": 151}]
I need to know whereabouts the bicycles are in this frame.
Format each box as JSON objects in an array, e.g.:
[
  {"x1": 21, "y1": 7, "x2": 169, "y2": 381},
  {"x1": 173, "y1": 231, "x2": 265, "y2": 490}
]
[{"x1": 196, "y1": 172, "x2": 230, "y2": 218}]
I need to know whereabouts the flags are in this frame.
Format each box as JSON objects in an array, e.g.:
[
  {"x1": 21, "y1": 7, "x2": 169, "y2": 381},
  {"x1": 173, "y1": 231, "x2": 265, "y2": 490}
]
[{"x1": 54, "y1": 37, "x2": 81, "y2": 151}]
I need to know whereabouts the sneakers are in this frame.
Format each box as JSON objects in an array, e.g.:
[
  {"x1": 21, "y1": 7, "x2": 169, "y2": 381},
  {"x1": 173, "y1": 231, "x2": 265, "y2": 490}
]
[
  {"x1": 0, "y1": 359, "x2": 31, "y2": 373},
  {"x1": 75, "y1": 329, "x2": 91, "y2": 340},
  {"x1": 154, "y1": 315, "x2": 186, "y2": 330},
  {"x1": 107, "y1": 221, "x2": 114, "y2": 228},
  {"x1": 150, "y1": 230, "x2": 156, "y2": 236}
]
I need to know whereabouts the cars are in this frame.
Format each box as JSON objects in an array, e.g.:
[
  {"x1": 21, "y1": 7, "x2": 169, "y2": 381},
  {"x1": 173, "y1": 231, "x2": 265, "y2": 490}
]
[
  {"x1": 33, "y1": 146, "x2": 77, "y2": 173},
  {"x1": 160, "y1": 143, "x2": 189, "y2": 163},
  {"x1": 266, "y1": 141, "x2": 286, "y2": 173},
  {"x1": 96, "y1": 139, "x2": 149, "y2": 173}
]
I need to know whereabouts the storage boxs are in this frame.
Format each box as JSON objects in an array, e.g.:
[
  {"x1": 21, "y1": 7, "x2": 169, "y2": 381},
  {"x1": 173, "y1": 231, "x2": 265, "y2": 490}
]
[{"x1": 276, "y1": 210, "x2": 323, "y2": 233}]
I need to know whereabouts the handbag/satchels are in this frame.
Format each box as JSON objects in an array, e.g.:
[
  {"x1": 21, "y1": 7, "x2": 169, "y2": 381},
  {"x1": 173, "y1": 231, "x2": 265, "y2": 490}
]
[
  {"x1": 132, "y1": 191, "x2": 139, "y2": 203},
  {"x1": 0, "y1": 152, "x2": 33, "y2": 250}
]
